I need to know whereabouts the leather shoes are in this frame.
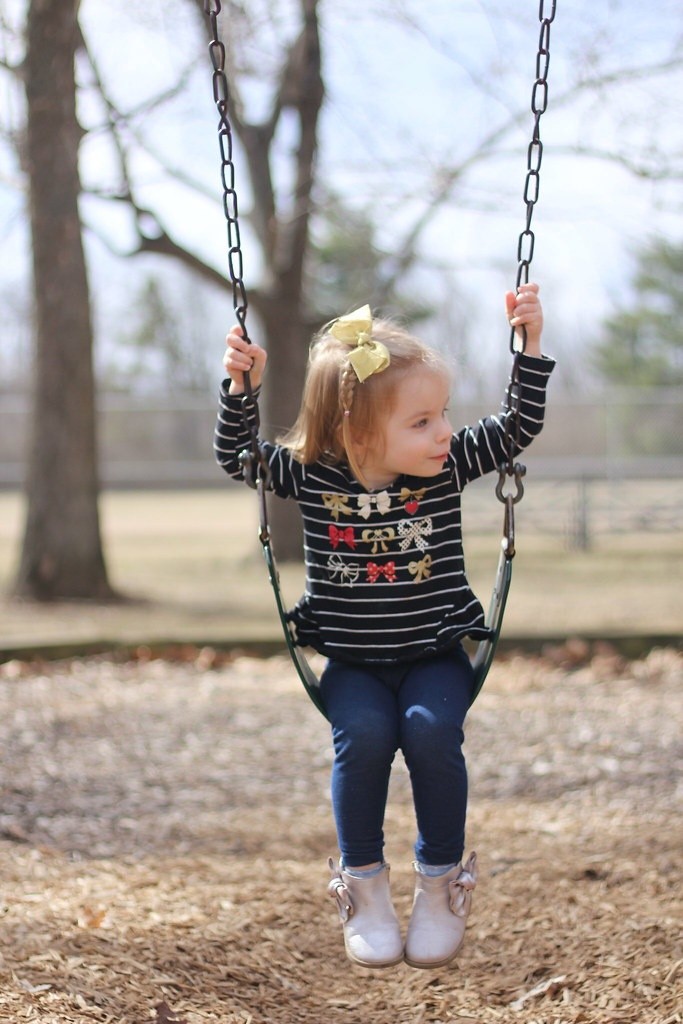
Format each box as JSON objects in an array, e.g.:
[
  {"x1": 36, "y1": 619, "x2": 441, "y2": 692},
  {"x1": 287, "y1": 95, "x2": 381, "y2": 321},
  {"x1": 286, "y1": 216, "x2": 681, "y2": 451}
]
[
  {"x1": 404, "y1": 851, "x2": 477, "y2": 969},
  {"x1": 327, "y1": 855, "x2": 404, "y2": 968}
]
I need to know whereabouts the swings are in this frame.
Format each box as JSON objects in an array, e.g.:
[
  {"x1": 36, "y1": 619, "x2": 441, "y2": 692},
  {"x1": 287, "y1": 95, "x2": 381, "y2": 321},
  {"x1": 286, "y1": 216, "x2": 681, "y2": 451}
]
[{"x1": 206, "y1": 0, "x2": 560, "y2": 728}]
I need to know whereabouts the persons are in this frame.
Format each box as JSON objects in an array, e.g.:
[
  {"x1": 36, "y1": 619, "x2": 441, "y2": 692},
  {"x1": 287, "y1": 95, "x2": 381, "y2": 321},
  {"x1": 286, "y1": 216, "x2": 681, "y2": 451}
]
[{"x1": 214, "y1": 282, "x2": 557, "y2": 969}]
